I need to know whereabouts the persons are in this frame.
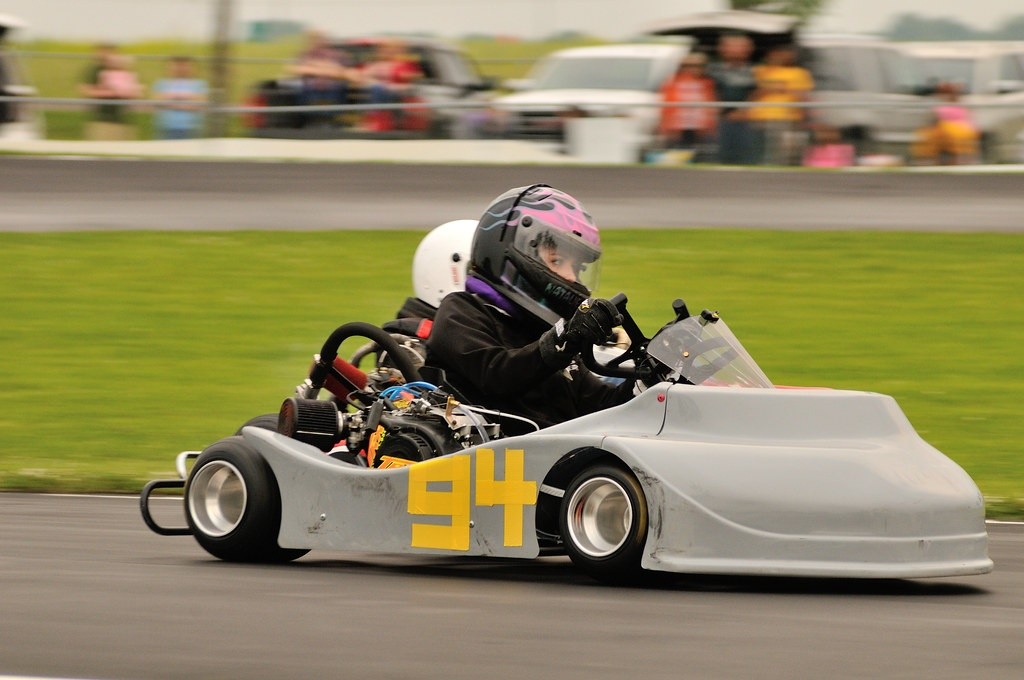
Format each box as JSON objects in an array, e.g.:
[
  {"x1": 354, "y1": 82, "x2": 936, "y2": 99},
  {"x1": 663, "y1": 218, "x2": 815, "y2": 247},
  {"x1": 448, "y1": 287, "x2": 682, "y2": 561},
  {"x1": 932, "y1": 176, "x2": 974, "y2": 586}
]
[
  {"x1": 375, "y1": 182, "x2": 647, "y2": 432},
  {"x1": 74, "y1": 33, "x2": 432, "y2": 139},
  {"x1": 651, "y1": 32, "x2": 815, "y2": 166}
]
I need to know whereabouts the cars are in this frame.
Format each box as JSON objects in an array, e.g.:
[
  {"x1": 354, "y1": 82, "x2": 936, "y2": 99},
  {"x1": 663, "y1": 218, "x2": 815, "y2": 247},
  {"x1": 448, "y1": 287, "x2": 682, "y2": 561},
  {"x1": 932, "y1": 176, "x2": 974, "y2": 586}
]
[
  {"x1": 798, "y1": 33, "x2": 1022, "y2": 163},
  {"x1": 247, "y1": 36, "x2": 507, "y2": 138},
  {"x1": 492, "y1": 44, "x2": 691, "y2": 141}
]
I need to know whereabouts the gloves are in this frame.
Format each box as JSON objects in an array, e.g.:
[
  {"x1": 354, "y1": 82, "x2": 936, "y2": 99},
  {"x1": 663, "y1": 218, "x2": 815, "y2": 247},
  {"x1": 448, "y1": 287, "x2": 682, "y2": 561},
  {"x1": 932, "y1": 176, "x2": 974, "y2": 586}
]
[{"x1": 563, "y1": 296, "x2": 625, "y2": 348}]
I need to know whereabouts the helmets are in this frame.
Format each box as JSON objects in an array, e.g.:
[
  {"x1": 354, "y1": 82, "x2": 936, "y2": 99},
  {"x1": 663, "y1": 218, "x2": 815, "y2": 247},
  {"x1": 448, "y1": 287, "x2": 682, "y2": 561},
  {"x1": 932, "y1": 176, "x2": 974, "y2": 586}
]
[
  {"x1": 464, "y1": 183, "x2": 603, "y2": 326},
  {"x1": 412, "y1": 218, "x2": 479, "y2": 311}
]
